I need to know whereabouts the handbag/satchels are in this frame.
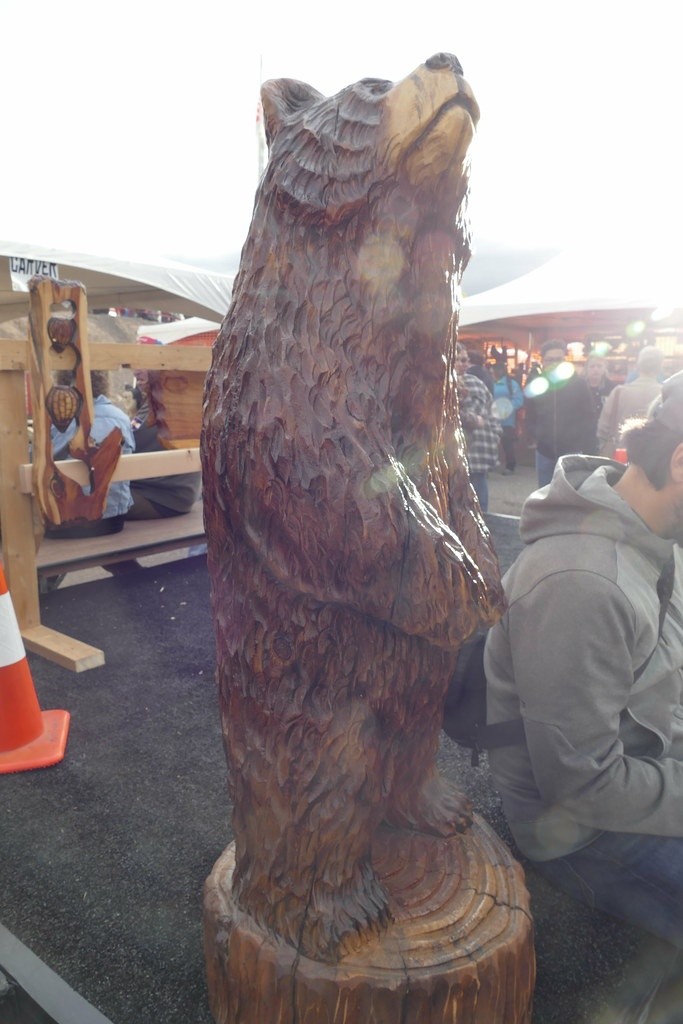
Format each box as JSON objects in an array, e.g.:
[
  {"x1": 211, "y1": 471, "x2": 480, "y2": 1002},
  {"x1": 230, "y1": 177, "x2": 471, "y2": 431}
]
[
  {"x1": 599, "y1": 388, "x2": 621, "y2": 457},
  {"x1": 443, "y1": 616, "x2": 488, "y2": 750},
  {"x1": 514, "y1": 407, "x2": 527, "y2": 440}
]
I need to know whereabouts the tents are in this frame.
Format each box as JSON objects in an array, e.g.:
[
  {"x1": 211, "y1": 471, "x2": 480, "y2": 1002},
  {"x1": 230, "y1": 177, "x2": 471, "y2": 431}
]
[
  {"x1": 456, "y1": 238, "x2": 683, "y2": 440},
  {"x1": 0, "y1": 238, "x2": 236, "y2": 344}
]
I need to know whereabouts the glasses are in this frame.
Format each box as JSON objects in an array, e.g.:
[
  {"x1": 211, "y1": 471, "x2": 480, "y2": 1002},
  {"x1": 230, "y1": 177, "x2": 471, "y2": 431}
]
[{"x1": 459, "y1": 356, "x2": 471, "y2": 364}]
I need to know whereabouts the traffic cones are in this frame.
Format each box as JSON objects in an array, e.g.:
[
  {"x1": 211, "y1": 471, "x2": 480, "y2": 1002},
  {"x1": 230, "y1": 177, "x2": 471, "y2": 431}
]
[{"x1": 1, "y1": 558, "x2": 73, "y2": 775}]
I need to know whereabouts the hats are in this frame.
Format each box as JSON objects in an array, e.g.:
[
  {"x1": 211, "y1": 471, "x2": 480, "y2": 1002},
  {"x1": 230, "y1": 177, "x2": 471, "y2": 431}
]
[
  {"x1": 539, "y1": 338, "x2": 568, "y2": 357},
  {"x1": 646, "y1": 369, "x2": 683, "y2": 434}
]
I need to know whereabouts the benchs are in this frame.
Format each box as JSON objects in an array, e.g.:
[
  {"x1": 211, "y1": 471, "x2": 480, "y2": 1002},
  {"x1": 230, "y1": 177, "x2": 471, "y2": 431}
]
[{"x1": 0, "y1": 448, "x2": 204, "y2": 579}]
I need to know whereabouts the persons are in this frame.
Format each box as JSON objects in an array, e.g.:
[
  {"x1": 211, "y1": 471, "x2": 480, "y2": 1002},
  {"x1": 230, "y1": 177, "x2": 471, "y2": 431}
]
[
  {"x1": 454, "y1": 339, "x2": 683, "y2": 512},
  {"x1": 484, "y1": 372, "x2": 683, "y2": 951},
  {"x1": 30, "y1": 369, "x2": 135, "y2": 594},
  {"x1": 125, "y1": 337, "x2": 202, "y2": 521}
]
